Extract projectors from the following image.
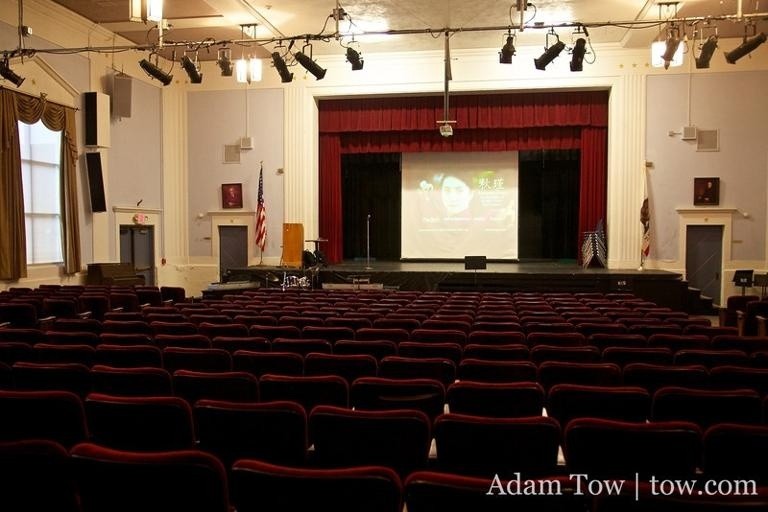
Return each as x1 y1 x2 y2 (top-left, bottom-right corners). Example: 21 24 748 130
440 125 454 136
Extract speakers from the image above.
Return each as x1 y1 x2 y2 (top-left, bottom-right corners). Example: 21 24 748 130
113 76 133 118
464 255 487 269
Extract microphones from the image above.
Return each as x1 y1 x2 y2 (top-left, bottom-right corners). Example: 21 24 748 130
366 214 370 223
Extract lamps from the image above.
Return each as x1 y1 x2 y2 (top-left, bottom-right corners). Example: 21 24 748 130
125 0 166 24
653 2 689 70
232 20 267 83
0 15 768 89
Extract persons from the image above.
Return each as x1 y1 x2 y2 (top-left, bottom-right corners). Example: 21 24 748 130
697 181 713 203
224 187 240 207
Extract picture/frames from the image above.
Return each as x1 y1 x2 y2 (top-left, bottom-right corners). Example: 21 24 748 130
692 175 721 207
221 182 244 211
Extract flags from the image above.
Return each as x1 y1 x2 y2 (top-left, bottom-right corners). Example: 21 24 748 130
638 164 651 264
253 164 266 252
